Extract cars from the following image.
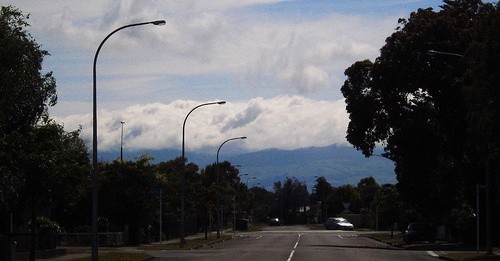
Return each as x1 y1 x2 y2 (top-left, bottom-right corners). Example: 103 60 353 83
325 217 354 231
269 218 281 227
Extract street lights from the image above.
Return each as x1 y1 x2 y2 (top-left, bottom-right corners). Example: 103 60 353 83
180 101 226 245
233 165 260 236
216 136 248 240
91 19 167 261
